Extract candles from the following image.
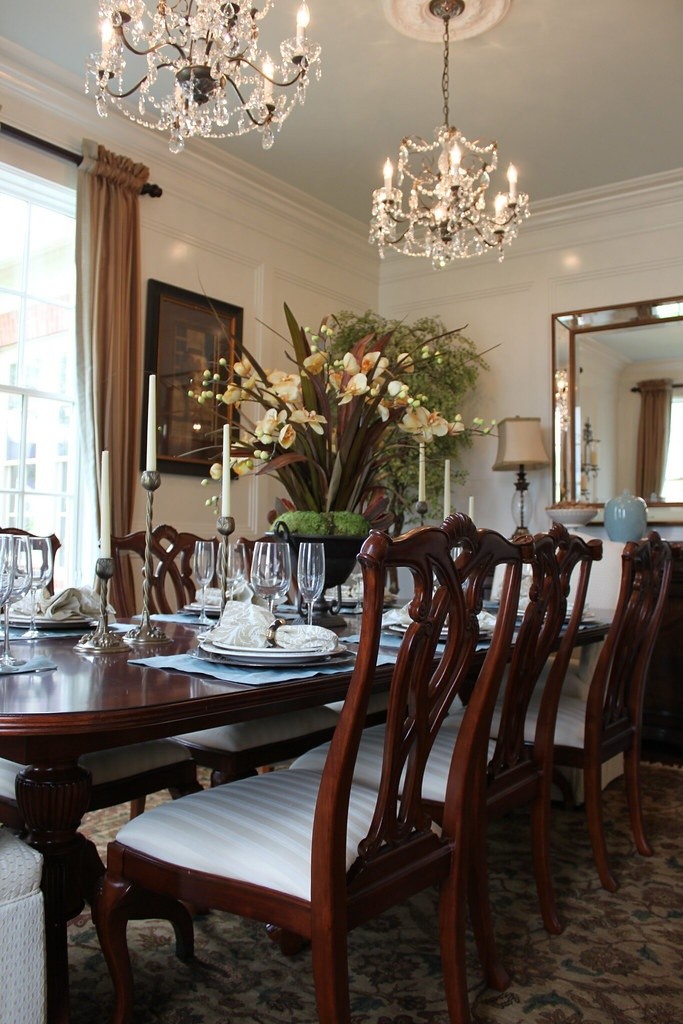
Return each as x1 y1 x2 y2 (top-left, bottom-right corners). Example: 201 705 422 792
220 423 233 519
101 450 113 560
443 459 453 521
468 496 474 523
145 375 157 475
418 440 428 506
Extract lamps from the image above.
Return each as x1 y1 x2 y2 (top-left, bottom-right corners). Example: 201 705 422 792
82 0 322 155
370 0 531 266
492 415 549 548
579 418 601 502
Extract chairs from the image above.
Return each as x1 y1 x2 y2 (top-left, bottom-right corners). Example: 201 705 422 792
293 524 601 996
236 532 390 728
101 515 515 1024
459 531 672 895
107 525 342 821
2 528 200 828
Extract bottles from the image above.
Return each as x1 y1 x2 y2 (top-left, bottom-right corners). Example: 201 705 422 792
603 489 649 543
250 541 291 613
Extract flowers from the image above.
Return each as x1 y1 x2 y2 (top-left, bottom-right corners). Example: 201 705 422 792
198 301 500 513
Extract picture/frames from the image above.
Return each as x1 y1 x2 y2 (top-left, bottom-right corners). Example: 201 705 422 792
142 278 243 482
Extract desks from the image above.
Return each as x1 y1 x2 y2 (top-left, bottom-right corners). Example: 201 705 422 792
0 598 615 1024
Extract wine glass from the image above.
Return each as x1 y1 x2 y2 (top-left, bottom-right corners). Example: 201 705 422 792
215 542 247 628
296 542 325 626
350 560 364 613
21 537 54 639
0 533 19 673
0 535 34 666
193 541 215 623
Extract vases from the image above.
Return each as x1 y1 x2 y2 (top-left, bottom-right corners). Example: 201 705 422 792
265 511 369 629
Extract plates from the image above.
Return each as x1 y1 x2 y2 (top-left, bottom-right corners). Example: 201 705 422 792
388 623 492 641
517 608 585 619
188 641 356 667
184 602 279 616
8 613 99 629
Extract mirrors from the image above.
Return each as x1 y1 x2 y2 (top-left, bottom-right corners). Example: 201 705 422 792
547 295 683 526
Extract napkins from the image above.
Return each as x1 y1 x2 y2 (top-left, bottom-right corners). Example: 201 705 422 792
209 599 336 652
13 588 115 623
196 582 255 614
500 572 591 621
381 595 496 632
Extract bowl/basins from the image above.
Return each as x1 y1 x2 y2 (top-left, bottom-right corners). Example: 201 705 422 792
545 509 598 528
